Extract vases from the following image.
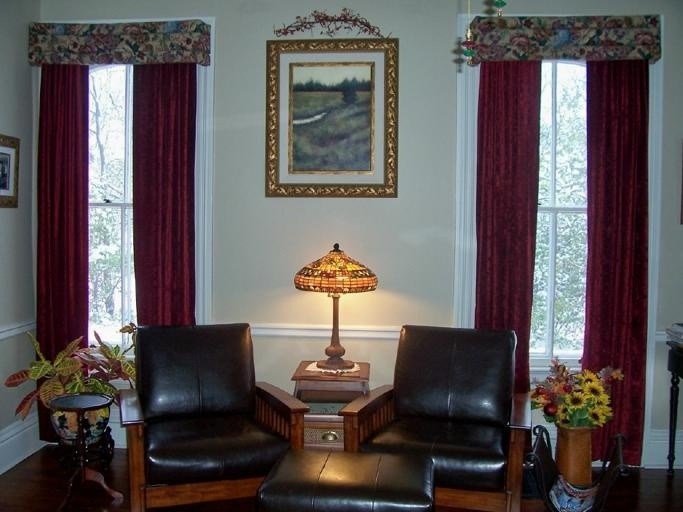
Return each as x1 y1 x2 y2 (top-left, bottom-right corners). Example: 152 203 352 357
555 425 593 486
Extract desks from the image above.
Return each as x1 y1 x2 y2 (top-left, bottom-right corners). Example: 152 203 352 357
665 342 683 474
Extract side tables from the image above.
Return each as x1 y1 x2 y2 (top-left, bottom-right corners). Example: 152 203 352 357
49 392 124 512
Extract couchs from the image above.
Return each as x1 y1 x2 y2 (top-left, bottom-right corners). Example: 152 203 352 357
337 325 532 512
257 448 434 512
118 323 310 512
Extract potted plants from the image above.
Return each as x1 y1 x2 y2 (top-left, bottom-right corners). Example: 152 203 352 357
3 321 136 450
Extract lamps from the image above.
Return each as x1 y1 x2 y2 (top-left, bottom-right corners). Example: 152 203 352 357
294 242 378 370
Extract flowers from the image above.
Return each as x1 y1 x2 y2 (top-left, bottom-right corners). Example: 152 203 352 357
527 356 625 427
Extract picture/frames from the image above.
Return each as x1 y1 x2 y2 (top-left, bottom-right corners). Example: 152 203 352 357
0 134 21 208
264 38 397 198
